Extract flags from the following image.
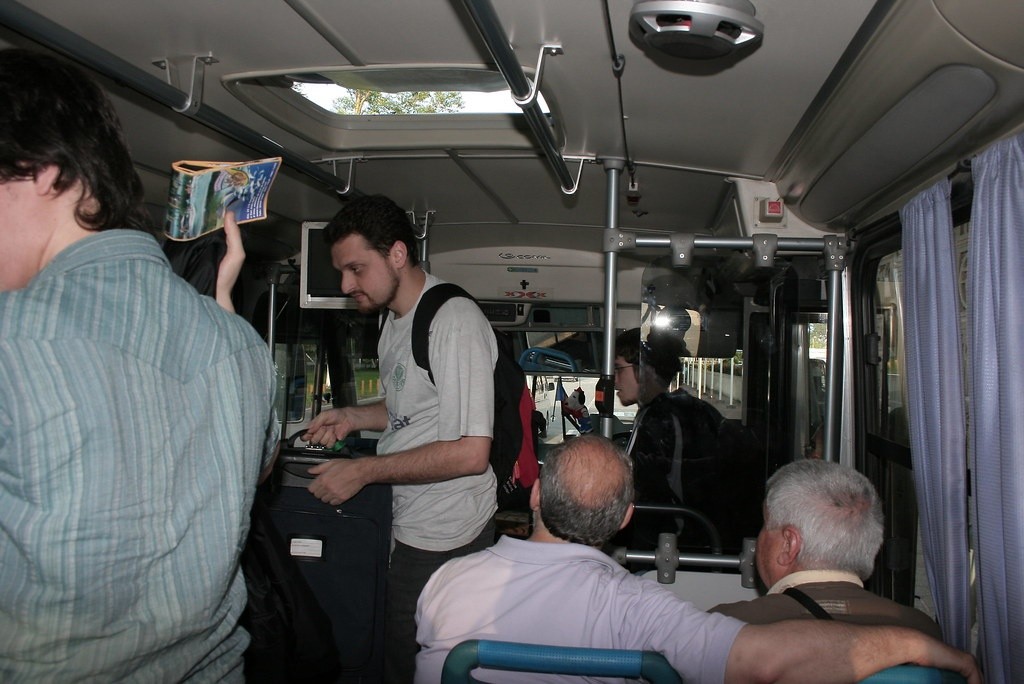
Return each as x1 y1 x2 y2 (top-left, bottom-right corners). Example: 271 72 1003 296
556 378 569 401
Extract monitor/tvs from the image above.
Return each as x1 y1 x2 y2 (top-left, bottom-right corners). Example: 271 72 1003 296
300 221 361 309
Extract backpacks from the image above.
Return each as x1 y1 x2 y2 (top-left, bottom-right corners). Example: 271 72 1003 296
374 283 547 487
636 390 765 555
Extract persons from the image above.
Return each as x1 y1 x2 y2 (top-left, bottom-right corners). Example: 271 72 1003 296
705 460 944 645
808 419 830 459
414 434 982 683
0 48 278 683
613 328 722 533
302 194 500 600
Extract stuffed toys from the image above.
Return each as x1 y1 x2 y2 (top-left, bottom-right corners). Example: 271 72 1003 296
562 386 593 432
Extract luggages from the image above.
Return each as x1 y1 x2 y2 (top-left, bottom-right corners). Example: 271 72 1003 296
242 429 391 684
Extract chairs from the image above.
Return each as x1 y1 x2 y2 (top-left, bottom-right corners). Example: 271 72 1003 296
519 346 576 372
441 640 971 683
